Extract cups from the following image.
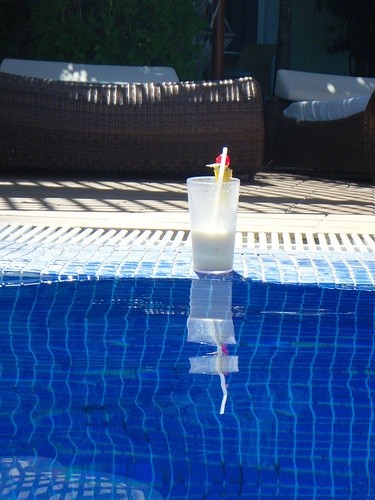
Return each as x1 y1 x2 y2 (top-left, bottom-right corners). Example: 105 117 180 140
187 175 241 275
185 280 242 379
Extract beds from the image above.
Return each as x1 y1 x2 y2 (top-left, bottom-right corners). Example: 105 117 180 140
267 70 373 181
1 57 262 183
